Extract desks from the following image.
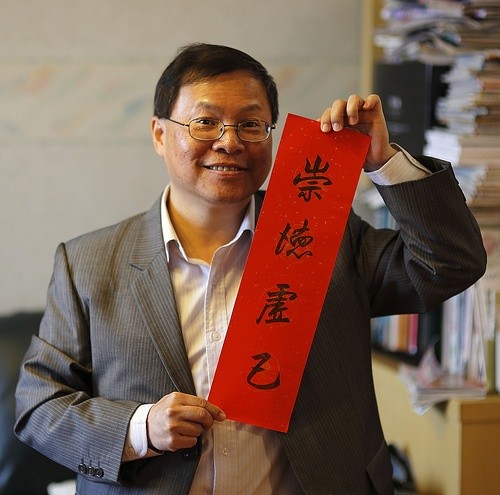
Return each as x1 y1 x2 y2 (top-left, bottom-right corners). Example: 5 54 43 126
363 344 500 495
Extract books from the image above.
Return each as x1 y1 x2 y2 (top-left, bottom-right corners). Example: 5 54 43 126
361 1 499 415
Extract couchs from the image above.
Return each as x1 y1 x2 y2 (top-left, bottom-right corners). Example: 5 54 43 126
1 310 79 495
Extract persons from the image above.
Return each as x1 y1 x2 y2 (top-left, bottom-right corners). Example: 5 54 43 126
13 44 487 495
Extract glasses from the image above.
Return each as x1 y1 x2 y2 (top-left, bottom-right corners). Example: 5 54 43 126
159 113 276 144
